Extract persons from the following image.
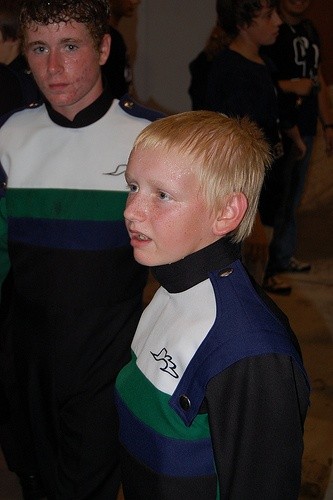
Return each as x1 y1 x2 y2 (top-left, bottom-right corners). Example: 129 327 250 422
0 0 169 500
189 0 327 294
112 109 311 500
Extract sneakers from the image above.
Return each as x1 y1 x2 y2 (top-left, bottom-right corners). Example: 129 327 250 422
267 256 311 272
262 270 292 295
